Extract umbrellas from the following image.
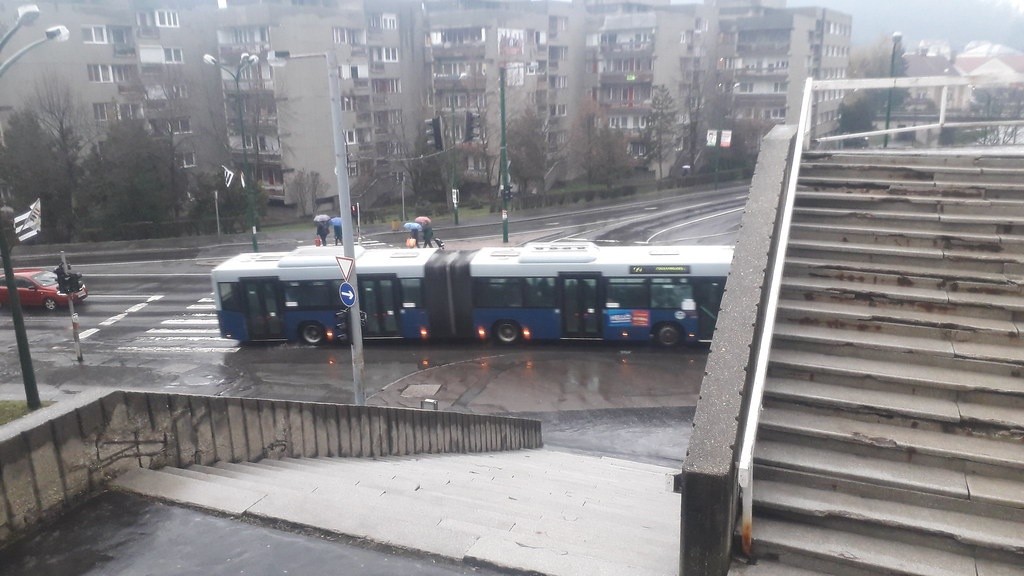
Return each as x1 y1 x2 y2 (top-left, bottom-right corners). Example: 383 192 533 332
415 216 431 226
329 218 342 226
314 214 330 222
403 223 422 231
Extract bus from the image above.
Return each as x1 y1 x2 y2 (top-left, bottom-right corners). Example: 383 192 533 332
211 241 736 347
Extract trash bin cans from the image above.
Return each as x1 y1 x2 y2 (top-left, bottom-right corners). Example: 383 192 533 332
392 220 400 231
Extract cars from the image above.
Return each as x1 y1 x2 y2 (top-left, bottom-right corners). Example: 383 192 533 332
0 270 88 312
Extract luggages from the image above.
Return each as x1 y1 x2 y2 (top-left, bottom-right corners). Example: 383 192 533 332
432 237 445 249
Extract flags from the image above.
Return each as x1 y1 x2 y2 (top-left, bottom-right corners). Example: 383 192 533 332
13 200 41 242
223 169 233 187
240 173 246 187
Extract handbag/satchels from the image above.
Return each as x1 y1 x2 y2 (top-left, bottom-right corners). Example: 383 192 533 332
406 237 417 247
316 236 320 246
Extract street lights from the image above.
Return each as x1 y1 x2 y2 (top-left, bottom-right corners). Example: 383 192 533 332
204 53 261 253
499 62 539 243
883 32 903 147
0 4 71 409
267 50 367 406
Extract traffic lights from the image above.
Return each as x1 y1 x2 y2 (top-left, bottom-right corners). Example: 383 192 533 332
53 268 72 294
70 273 83 292
466 111 480 141
424 117 442 150
508 185 513 201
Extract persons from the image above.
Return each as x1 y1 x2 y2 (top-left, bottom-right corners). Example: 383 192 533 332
707 133 715 146
317 221 330 246
422 223 433 248
410 229 420 248
334 225 343 246
